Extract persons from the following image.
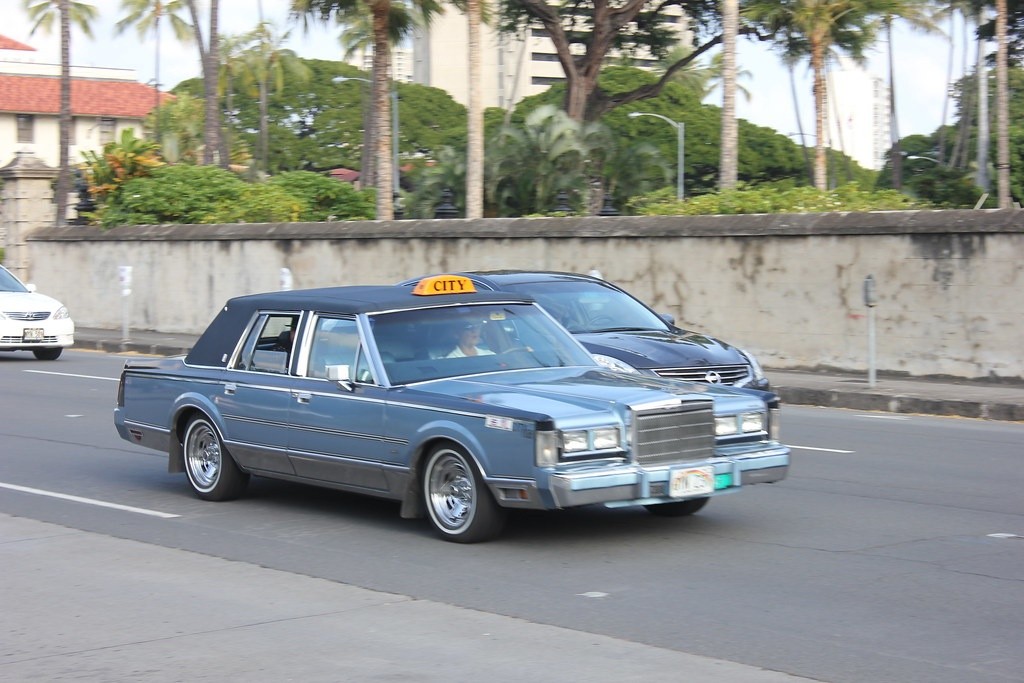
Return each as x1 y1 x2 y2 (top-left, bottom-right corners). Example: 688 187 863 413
445 316 495 358
273 314 323 373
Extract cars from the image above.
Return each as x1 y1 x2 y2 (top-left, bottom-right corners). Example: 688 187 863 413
0 263 76 359
327 266 771 394
114 271 790 544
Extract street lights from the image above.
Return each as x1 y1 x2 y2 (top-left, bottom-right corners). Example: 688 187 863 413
332 75 402 221
626 112 685 207
905 154 944 204
787 131 837 192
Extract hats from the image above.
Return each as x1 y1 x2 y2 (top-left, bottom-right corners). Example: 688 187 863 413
284 315 327 332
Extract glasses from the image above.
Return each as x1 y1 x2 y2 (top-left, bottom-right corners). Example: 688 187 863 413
462 320 487 331
